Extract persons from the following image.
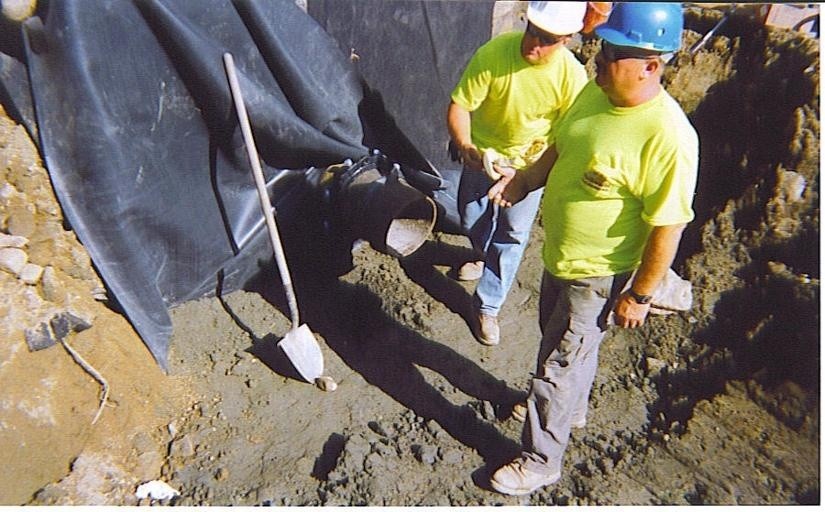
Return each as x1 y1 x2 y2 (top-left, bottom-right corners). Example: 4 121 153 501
443 1 589 347
489 0 698 495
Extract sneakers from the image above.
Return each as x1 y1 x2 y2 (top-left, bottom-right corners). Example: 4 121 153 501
487 457 561 495
511 401 586 428
464 313 499 345
458 260 485 281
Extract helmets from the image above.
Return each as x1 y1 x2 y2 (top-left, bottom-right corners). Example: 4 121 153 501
593 2 684 52
527 1 587 35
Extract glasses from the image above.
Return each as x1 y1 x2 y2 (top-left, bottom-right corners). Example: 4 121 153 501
528 23 561 45
602 39 651 61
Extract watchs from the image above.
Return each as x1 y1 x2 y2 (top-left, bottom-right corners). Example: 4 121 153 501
630 288 654 308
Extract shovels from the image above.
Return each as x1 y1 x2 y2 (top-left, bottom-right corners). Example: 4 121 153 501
223 52 324 386
